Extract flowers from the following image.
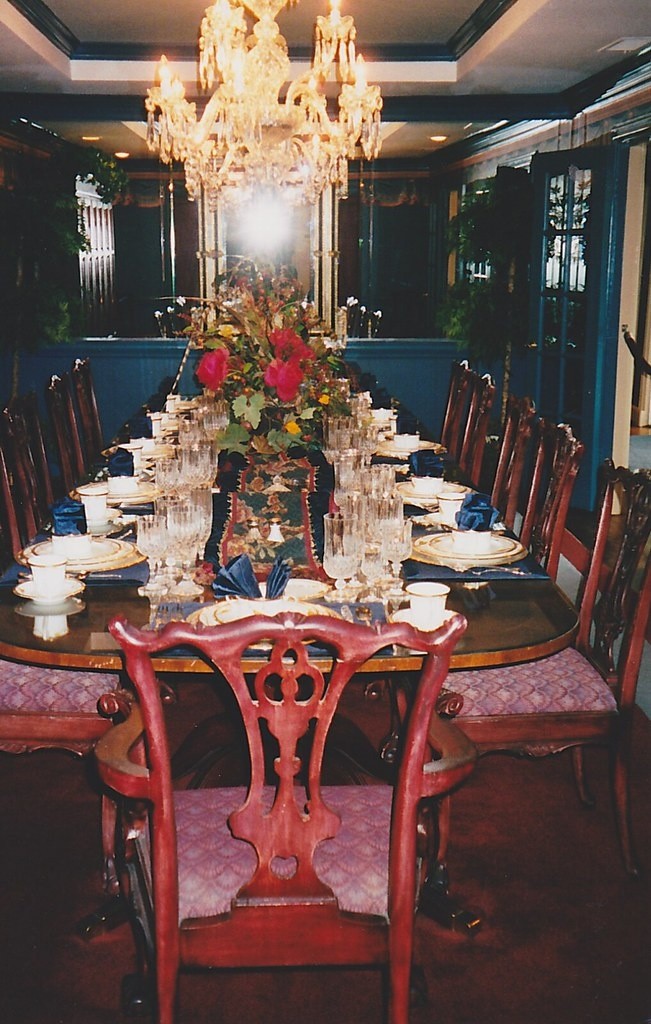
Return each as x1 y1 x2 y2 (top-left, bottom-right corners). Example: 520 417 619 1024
148 249 383 454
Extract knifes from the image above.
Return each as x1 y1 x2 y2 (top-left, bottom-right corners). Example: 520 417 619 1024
342 605 354 624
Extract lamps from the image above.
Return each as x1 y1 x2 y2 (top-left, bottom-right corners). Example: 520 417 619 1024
146 0 383 213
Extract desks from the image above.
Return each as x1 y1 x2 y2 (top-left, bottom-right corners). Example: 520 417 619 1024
0 393 579 672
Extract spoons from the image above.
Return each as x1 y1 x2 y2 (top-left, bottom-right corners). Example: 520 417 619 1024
356 606 373 627
18 570 90 580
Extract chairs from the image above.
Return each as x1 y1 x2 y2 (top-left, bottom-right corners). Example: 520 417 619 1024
0 356 651 1024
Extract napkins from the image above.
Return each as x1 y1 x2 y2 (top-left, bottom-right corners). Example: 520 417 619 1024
212 553 292 602
107 448 134 475
149 602 394 658
457 491 498 530
402 527 551 579
49 493 87 536
0 534 149 587
409 449 454 476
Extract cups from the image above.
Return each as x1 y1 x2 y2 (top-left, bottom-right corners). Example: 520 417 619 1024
130 439 156 454
108 476 139 493
27 555 67 595
51 533 92 560
452 530 491 553
166 394 176 412
436 493 466 521
412 478 445 494
79 488 109 519
151 414 162 437
406 582 450 623
370 410 393 419
394 435 420 449
118 444 143 466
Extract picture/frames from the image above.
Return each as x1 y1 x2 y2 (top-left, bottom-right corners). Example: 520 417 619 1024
197 179 338 335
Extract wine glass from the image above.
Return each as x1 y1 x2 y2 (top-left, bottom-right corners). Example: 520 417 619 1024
333 427 395 507
153 495 182 577
136 516 167 596
166 504 205 595
367 491 404 579
344 494 362 586
155 409 230 493
191 488 212 566
382 519 413 600
323 513 358 603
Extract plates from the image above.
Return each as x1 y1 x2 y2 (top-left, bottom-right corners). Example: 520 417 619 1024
171 401 196 410
76 510 123 525
188 599 341 649
252 579 328 601
378 440 443 457
389 608 468 632
409 533 526 567
100 443 176 458
70 479 157 506
15 537 147 570
397 481 471 505
13 580 86 603
